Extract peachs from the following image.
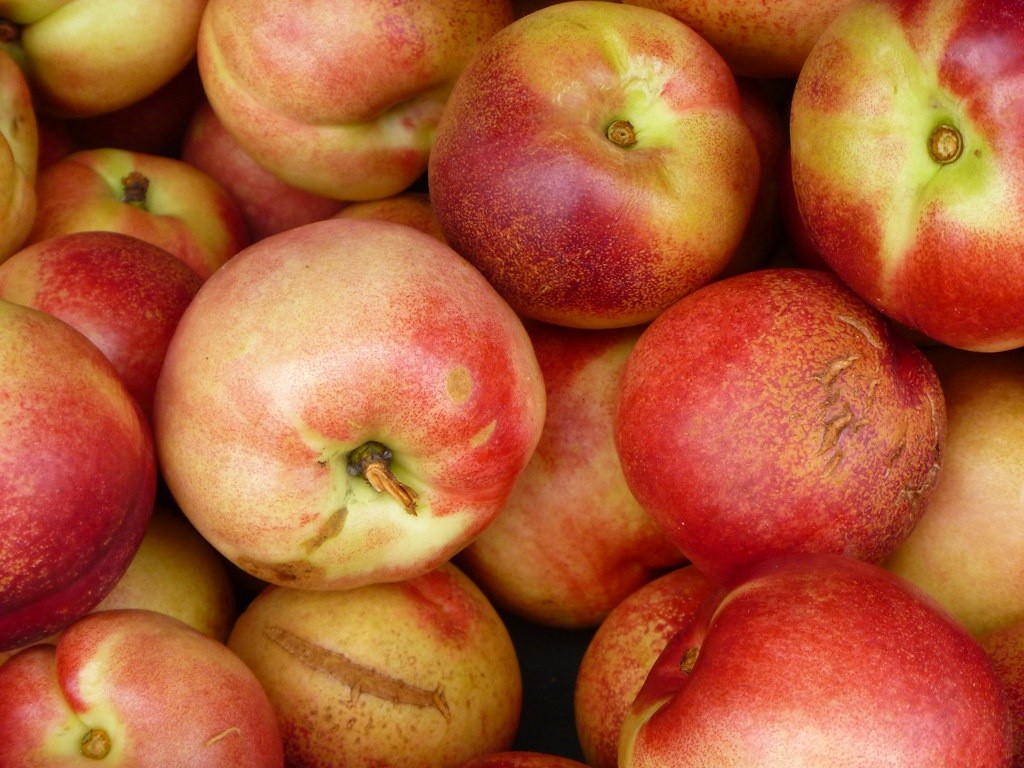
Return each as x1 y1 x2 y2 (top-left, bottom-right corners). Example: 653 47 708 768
0 0 1024 768
